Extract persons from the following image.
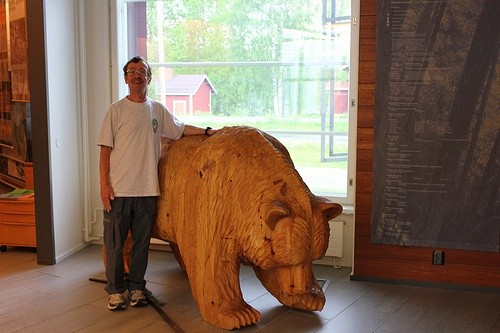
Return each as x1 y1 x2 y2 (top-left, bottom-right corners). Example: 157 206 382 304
96 55 222 311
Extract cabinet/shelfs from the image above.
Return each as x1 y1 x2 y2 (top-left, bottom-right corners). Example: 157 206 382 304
0 189 37 252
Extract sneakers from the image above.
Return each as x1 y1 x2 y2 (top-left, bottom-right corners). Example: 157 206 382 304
108 293 128 311
128 290 148 307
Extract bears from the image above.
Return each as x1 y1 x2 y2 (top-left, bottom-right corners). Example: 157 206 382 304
102 125 343 330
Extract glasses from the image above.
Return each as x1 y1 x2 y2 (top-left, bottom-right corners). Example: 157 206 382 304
126 70 149 74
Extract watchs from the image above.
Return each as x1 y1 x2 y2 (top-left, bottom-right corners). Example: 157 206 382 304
205 126 212 136
